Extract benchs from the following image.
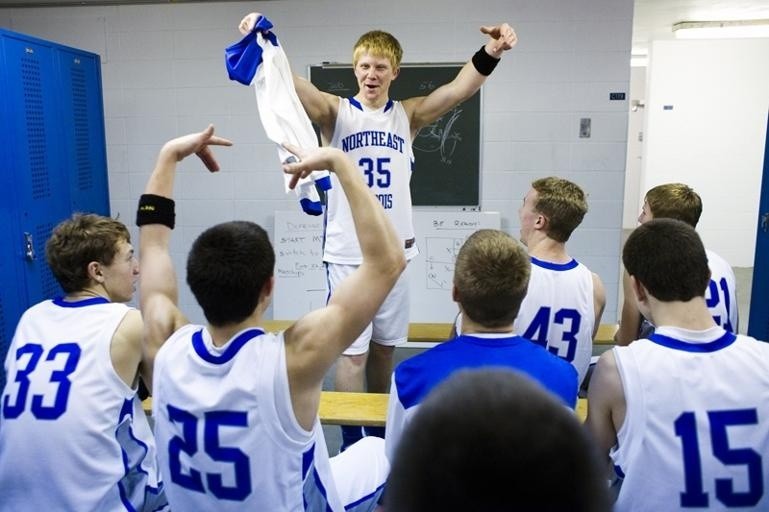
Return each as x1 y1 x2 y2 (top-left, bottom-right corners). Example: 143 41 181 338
141 391 590 428
257 316 621 345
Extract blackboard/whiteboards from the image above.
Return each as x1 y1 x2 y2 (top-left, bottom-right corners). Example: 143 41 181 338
273 210 501 323
307 62 483 211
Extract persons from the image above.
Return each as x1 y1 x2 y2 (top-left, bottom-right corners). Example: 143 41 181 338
237 11 518 451
385 228 579 466
578 182 739 398
0 208 172 512
449 177 606 402
382 365 615 512
584 217 769 512
134 124 408 512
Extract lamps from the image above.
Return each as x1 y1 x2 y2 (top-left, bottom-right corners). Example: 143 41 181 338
671 19 769 39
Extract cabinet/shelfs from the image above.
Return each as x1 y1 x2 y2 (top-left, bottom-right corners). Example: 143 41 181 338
0 30 112 396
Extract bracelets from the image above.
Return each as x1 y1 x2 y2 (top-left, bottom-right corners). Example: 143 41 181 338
471 44 502 76
136 193 176 229
